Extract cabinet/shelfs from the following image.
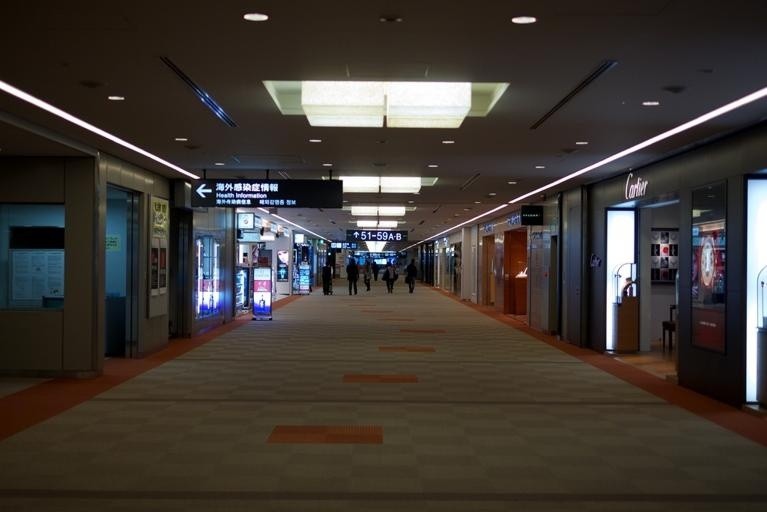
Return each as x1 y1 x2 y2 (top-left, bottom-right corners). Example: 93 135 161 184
610 260 642 353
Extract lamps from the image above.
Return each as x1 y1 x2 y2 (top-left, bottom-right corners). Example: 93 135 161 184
339 174 422 194
351 205 406 217
301 79 473 128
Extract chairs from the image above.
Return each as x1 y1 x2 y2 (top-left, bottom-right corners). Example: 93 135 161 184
661 304 677 353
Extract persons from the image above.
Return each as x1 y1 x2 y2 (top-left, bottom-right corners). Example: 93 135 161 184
381 262 396 294
363 262 370 291
322 261 333 295
403 258 417 293
356 261 402 277
372 262 378 281
346 259 359 295
277 251 287 265
